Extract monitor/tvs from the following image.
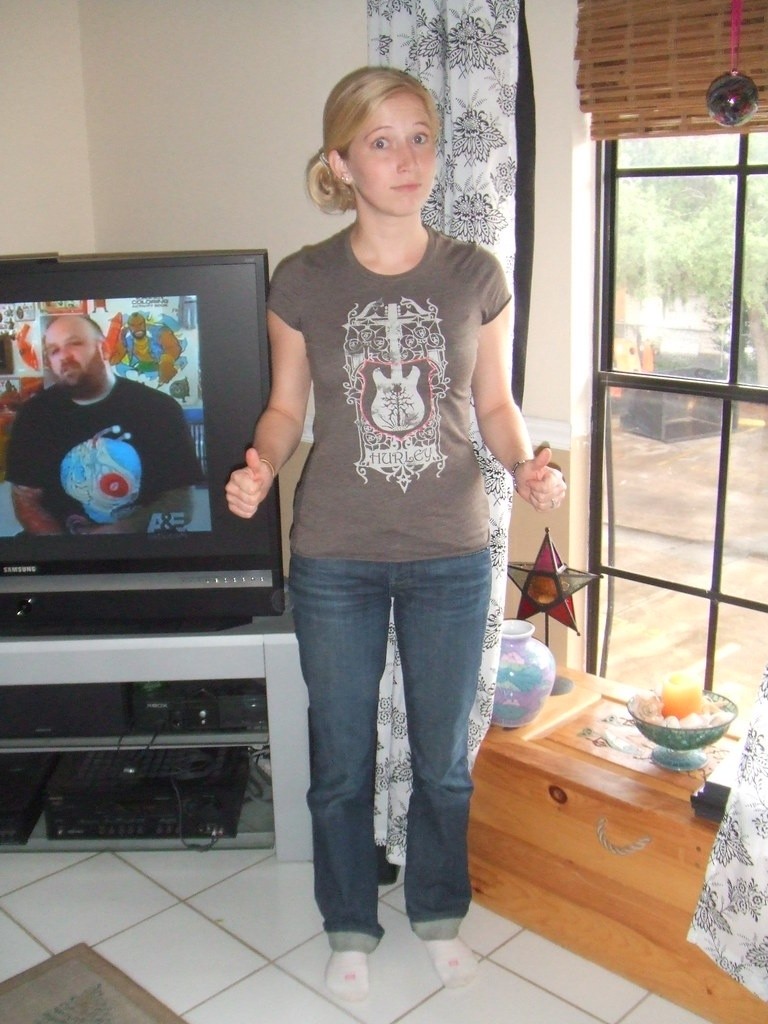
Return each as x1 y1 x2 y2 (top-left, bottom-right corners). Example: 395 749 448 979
0 249 287 637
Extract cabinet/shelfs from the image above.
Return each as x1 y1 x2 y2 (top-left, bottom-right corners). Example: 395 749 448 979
0 581 313 862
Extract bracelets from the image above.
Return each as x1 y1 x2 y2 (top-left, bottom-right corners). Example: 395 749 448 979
511 459 526 493
258 458 275 477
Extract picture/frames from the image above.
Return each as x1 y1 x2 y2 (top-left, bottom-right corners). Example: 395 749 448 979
43 300 86 313
0 333 14 375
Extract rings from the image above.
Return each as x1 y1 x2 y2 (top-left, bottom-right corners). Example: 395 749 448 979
551 498 559 508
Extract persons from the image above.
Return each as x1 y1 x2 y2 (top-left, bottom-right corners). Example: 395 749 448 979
224 66 572 996
4 314 201 539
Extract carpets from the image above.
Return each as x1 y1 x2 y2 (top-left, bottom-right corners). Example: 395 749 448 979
0 943 189 1024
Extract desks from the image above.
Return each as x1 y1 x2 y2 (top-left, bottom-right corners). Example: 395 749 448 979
468 665 768 1024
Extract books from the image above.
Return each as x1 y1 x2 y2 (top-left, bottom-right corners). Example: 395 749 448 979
689 734 747 824
